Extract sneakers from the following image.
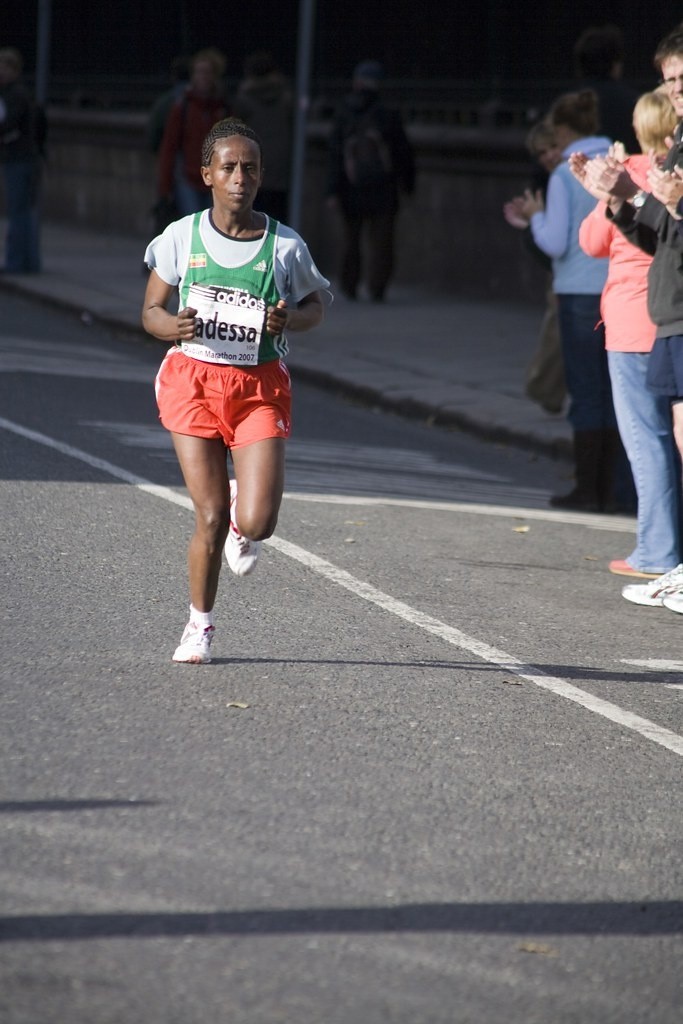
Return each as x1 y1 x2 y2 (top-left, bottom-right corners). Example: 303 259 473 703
171 620 216 666
663 589 683 613
225 478 260 576
621 564 683 606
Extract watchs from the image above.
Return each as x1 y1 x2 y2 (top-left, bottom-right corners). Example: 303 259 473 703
631 191 649 210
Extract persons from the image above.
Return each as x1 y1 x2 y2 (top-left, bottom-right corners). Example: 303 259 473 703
0 48 50 275
502 23 683 614
141 117 330 664
142 47 235 273
324 62 418 303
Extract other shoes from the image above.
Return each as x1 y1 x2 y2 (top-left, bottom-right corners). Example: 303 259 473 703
609 560 664 578
547 488 637 514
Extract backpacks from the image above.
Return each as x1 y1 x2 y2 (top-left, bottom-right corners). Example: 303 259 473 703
339 108 398 190
